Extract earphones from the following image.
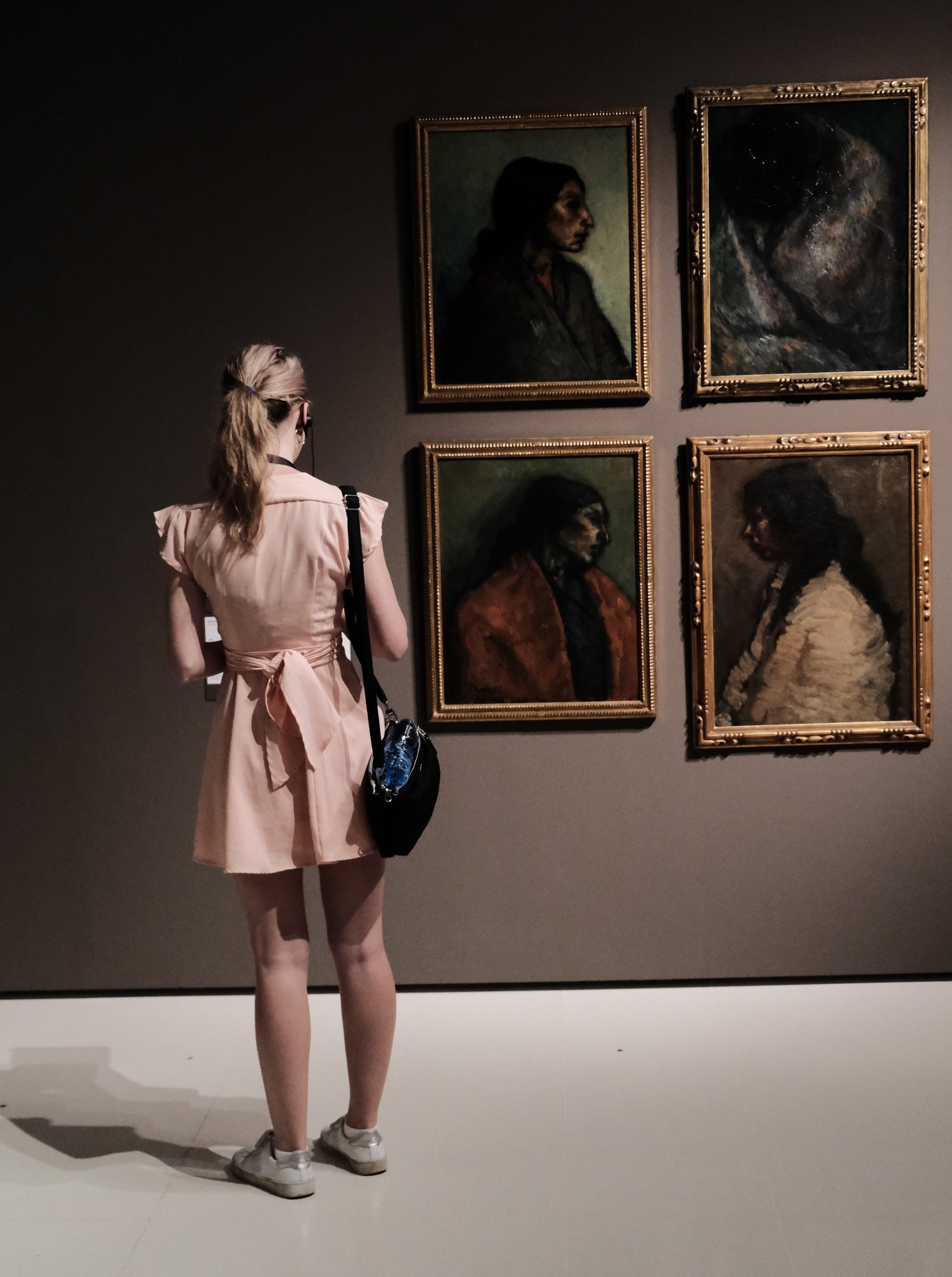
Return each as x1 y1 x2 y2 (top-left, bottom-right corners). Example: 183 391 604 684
304 414 313 430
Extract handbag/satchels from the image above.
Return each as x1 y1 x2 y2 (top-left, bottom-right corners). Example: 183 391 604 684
361 718 442 859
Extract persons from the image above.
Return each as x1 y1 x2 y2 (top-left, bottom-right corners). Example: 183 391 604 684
152 344 409 1201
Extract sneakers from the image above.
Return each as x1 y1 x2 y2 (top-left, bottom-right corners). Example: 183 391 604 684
321 1113 387 1176
232 1129 317 1198
